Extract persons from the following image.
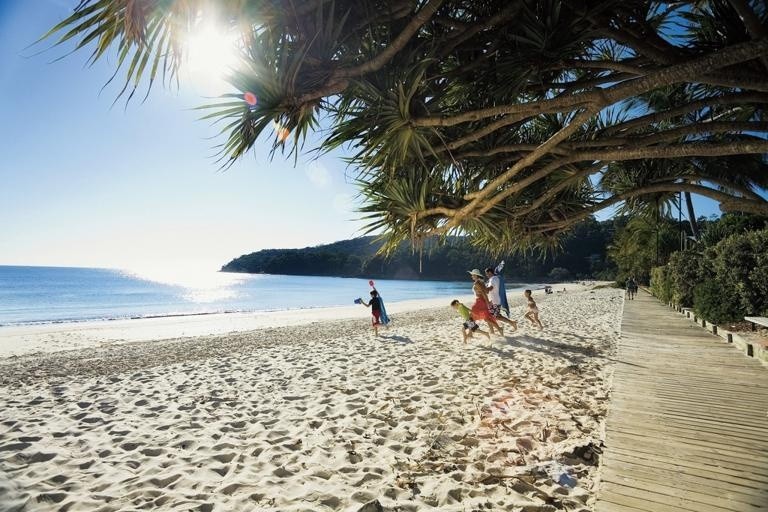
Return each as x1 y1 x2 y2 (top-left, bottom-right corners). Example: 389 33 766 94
467 269 504 337
359 290 388 337
451 300 490 345
484 268 518 334
524 290 543 331
634 279 638 293
632 276 635 284
626 277 635 300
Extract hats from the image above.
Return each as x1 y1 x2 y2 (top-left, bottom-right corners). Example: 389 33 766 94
466 268 485 279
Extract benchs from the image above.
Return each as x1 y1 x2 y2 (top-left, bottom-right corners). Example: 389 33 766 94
743 316 768 332
